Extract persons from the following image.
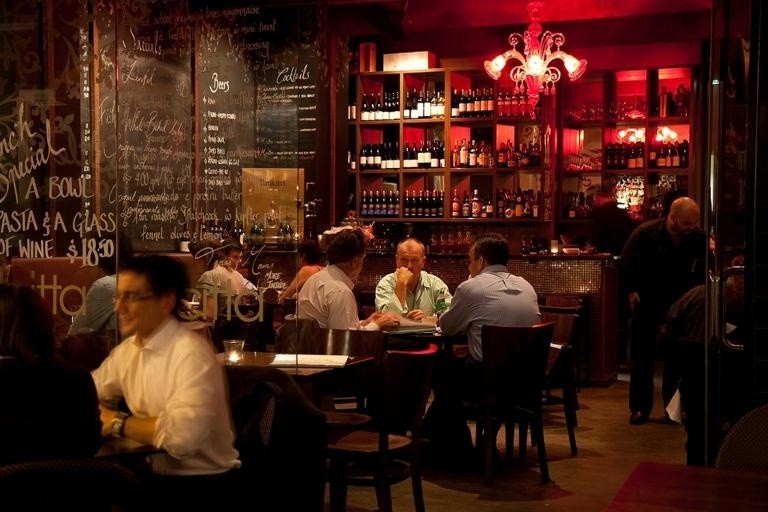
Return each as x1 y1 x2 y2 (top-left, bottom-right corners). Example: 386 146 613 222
195 240 267 352
665 247 744 466
582 185 642 372
618 198 715 426
0 283 105 467
437 234 541 476
280 240 326 299
297 228 400 395
375 239 453 436
90 256 253 512
0 252 11 285
67 230 135 370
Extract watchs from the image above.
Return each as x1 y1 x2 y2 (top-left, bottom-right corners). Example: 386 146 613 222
111 412 129 437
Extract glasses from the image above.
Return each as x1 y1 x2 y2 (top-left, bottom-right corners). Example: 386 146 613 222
111 289 151 304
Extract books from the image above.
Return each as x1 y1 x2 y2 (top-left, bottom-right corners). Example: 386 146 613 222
270 354 356 368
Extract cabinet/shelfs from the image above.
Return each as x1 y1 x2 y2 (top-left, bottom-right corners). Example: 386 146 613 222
355 72 550 253
552 67 701 253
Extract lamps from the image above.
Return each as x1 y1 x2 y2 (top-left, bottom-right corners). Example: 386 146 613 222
618 93 678 145
484 17 588 121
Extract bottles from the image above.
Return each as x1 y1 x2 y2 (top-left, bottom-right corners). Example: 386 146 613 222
451 88 459 117
368 93 375 121
438 189 444 218
387 190 394 218
435 288 447 319
430 89 438 120
394 91 400 120
473 88 480 119
656 140 665 167
439 140 446 169
628 142 637 168
374 190 380 218
512 91 518 117
424 90 431 120
496 139 542 169
480 88 488 117
437 91 444 119
679 140 688 168
404 90 410 119
613 142 620 170
387 142 394 170
672 141 680 168
519 91 526 117
636 141 644 168
380 190 387 219
403 141 410 169
466 89 474 118
620 142 627 169
410 189 417 218
665 141 672 167
417 90 424 119
459 138 467 168
424 140 431 169
394 189 400 218
417 140 424 168
471 188 480 219
380 143 386 170
417 189 424 218
423 189 430 218
351 105 356 120
504 90 512 117
411 142 418 169
459 89 467 119
478 141 488 168
375 93 382 120
451 139 459 169
360 94 368 120
389 93 395 120
367 190 374 219
487 87 494 118
497 91 503 117
361 190 367 218
366 143 374 170
394 140 400 170
461 190 471 219
451 188 460 218
346 105 351 119
496 186 542 219
429 189 437 218
486 190 494 219
410 88 419 120
382 92 389 120
374 143 381 169
649 141 656 167
403 189 410 218
468 140 477 168
431 142 438 168
359 142 366 170
605 142 613 170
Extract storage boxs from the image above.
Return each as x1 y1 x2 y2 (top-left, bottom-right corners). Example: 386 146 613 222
383 50 436 73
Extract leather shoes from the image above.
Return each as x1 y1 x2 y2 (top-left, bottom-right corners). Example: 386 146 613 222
629 410 648 424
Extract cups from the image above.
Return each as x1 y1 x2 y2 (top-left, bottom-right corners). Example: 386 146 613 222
222 340 245 368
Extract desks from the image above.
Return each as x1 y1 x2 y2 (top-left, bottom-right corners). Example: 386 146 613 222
215 345 378 410
380 324 467 358
603 460 768 512
91 404 168 512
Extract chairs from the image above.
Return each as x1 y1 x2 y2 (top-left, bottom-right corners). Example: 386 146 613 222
0 461 149 511
325 343 440 512
312 327 383 429
502 333 593 471
441 321 555 484
